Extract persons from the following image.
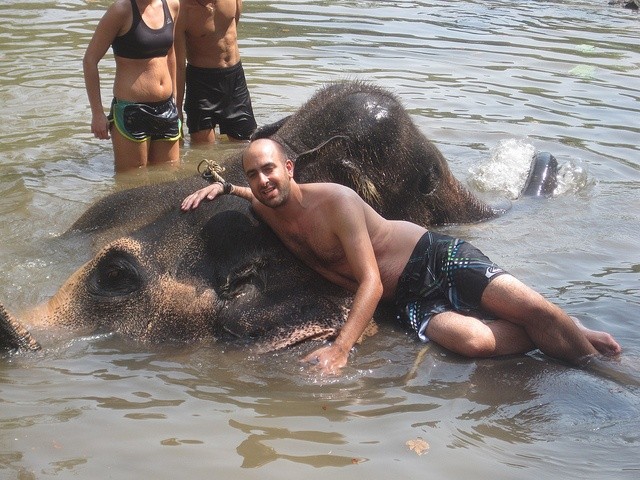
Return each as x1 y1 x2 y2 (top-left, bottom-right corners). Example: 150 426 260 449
82 1 181 169
175 0 257 143
181 139 622 379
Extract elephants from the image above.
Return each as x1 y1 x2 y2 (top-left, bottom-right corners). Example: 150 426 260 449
3 80 559 372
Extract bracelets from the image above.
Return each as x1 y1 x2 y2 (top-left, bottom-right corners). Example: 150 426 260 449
223 181 231 195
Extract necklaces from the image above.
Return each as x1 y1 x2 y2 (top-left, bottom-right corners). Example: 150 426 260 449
197 0 215 14
